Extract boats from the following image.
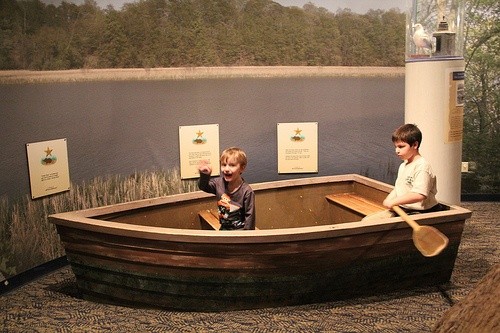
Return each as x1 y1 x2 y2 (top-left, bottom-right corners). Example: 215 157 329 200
46 172 473 311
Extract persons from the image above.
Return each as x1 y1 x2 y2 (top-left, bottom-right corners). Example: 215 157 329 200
359 123 440 222
198 147 256 230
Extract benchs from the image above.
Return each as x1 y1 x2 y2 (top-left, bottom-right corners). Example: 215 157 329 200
199 209 260 231
325 194 390 216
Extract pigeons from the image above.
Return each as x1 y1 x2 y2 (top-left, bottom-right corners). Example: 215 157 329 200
412 24 433 55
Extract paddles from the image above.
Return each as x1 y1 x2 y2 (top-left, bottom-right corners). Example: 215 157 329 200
391 204 449 258
